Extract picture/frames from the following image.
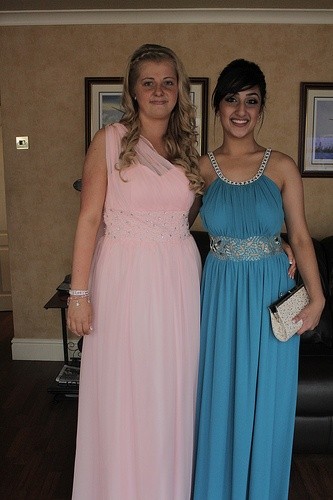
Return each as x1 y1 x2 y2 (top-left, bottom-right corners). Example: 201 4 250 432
298 81 333 178
85 76 209 159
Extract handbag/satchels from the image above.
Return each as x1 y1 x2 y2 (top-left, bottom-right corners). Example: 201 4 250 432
267 282 310 343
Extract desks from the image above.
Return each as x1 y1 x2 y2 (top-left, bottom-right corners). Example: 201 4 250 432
44 273 83 400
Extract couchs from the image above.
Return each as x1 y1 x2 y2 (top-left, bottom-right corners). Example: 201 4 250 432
191 231 333 452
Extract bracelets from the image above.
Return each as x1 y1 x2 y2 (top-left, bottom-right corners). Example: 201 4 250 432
67 289 91 307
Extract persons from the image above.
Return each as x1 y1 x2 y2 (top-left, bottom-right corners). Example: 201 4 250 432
187 58 326 500
66 44 298 500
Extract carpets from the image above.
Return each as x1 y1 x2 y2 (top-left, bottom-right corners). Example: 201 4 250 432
296 455 333 500
